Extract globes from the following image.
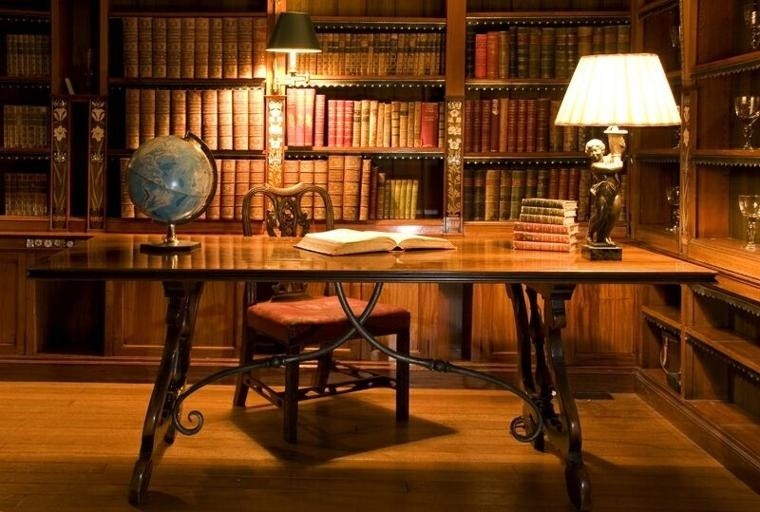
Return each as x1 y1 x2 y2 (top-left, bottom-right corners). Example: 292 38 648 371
125 130 218 252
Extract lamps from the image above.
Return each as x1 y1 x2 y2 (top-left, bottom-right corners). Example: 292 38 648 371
265 13 322 80
554 52 681 260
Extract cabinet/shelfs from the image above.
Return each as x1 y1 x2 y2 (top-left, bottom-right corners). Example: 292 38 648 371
636 0 760 497
1 0 638 394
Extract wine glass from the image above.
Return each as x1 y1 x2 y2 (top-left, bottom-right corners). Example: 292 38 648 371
734 95 760 150
669 25 679 69
672 125 680 150
742 3 759 50
737 195 759 251
666 185 680 233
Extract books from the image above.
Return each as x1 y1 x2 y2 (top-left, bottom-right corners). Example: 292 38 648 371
122 17 267 223
512 196 579 253
294 226 457 256
284 2 445 222
3 106 50 152
464 1 633 220
5 35 51 78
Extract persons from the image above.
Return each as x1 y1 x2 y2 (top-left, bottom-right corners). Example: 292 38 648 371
4 173 48 215
580 138 626 248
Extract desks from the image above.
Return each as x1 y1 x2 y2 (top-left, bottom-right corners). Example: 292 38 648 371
27 233 718 512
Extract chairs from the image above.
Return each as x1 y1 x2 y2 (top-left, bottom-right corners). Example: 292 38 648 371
234 183 410 443
